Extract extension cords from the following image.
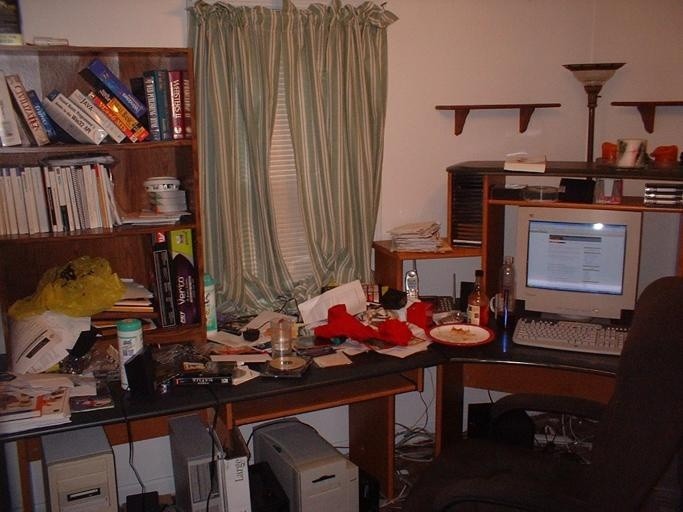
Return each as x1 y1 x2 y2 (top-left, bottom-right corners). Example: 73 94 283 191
534 434 575 447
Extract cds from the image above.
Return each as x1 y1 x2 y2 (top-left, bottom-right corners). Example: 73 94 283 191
526 186 558 201
270 356 307 370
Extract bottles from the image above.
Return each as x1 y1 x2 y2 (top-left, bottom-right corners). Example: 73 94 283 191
269 317 293 359
498 256 516 327
202 274 218 338
116 318 143 392
466 270 491 327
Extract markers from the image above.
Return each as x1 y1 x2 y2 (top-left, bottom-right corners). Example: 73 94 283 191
175 376 232 385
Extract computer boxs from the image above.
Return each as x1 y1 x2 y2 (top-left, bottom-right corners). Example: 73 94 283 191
39 426 119 511
253 417 360 512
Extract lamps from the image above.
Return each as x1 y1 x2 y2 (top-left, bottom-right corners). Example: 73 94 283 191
563 63 627 180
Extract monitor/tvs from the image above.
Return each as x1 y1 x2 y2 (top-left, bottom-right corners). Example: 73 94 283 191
514 204 643 321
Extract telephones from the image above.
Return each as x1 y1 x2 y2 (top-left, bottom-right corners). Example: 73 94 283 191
405 259 456 312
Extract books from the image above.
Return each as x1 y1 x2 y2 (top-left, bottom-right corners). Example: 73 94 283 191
91 229 195 329
1 153 192 236
1 58 191 146
502 152 548 174
0 371 114 435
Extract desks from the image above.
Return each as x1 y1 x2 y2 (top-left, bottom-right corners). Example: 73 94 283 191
427 318 621 458
0 345 450 512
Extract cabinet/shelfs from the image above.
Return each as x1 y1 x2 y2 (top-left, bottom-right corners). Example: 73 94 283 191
371 161 683 314
0 45 207 370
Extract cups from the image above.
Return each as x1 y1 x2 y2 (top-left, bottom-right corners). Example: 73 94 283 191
489 294 513 320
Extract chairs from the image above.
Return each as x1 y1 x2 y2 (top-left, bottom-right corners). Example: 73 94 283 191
421 275 683 512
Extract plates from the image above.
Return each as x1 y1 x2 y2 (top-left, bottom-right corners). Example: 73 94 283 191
425 323 496 346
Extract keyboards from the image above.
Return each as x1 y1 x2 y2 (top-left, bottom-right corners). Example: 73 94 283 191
512 317 631 357
